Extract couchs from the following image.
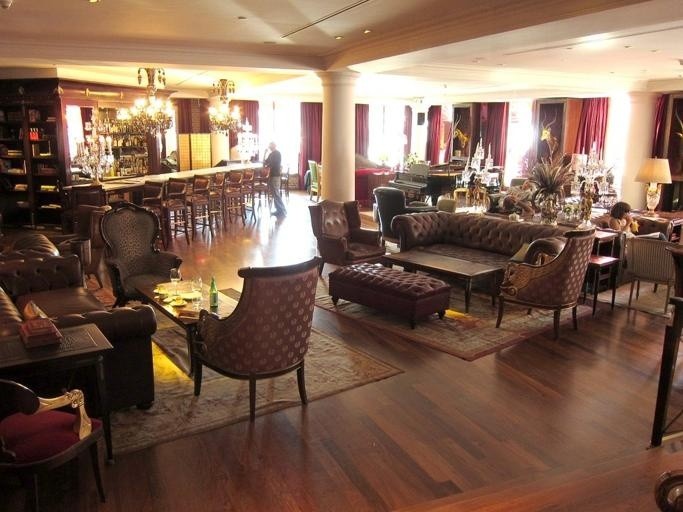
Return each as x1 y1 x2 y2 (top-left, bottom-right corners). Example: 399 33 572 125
390 209 565 269
1 232 158 411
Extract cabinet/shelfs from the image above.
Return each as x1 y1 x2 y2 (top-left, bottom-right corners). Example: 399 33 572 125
95 107 149 176
0 77 64 226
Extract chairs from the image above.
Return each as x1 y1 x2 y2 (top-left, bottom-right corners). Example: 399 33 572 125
0 378 106 512
624 238 676 316
495 228 595 335
308 158 321 203
308 201 386 265
62 168 290 251
372 187 438 246
193 255 323 423
99 202 184 308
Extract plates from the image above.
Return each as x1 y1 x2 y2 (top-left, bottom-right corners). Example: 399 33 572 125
152 289 196 306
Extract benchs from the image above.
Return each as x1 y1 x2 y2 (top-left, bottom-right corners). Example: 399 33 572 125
329 262 451 330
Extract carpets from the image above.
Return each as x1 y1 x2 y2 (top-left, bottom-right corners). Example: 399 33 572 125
88 270 404 454
314 290 599 358
587 272 679 325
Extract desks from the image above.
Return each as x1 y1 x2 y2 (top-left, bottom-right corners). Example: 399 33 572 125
380 250 502 313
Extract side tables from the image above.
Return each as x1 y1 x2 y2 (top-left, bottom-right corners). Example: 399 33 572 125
1 323 115 461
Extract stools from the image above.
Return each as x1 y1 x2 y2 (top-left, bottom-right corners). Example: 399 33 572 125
589 251 621 317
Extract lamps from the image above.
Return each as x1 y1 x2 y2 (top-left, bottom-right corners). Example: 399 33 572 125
208 78 241 130
634 156 673 215
578 139 607 190
236 118 259 162
73 106 114 183
115 67 178 134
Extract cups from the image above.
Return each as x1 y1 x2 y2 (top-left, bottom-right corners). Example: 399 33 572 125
508 214 519 221
532 214 541 223
455 150 462 158
631 222 638 231
116 171 121 177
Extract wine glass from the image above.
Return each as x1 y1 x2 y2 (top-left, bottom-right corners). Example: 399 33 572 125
189 276 203 311
169 268 181 292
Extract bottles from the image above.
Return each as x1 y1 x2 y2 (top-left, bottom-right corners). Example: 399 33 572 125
110 167 114 177
209 276 218 308
108 119 144 148
28 127 44 140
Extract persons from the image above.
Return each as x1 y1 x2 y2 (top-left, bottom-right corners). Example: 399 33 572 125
607 202 668 247
264 142 287 216
499 179 532 201
498 196 535 218
